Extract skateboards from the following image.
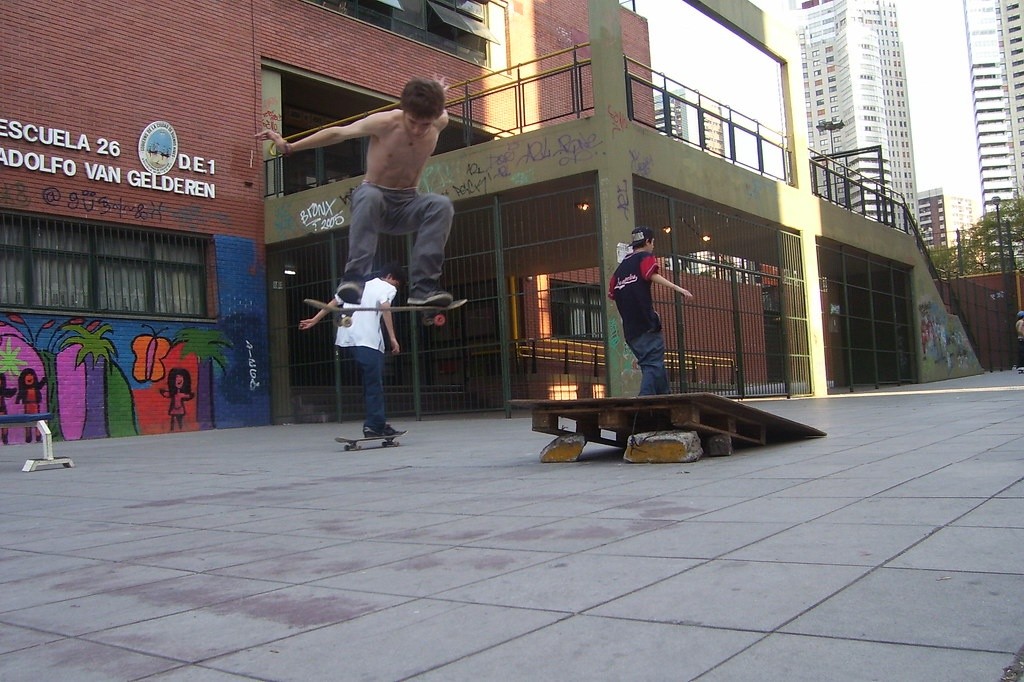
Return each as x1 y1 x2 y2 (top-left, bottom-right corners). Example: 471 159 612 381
334 430 409 451
1017 369 1024 374
304 298 468 328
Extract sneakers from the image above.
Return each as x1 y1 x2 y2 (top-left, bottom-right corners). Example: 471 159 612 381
363 424 385 438
337 274 365 305
407 278 453 307
384 424 399 435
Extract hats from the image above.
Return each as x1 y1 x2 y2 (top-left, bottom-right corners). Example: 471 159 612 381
627 225 651 247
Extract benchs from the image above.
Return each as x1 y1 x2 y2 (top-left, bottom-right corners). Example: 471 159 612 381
0 413 73 473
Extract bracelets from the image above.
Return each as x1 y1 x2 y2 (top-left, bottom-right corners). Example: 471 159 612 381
284 142 292 156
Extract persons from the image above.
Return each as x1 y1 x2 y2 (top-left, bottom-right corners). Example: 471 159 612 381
607 227 692 396
299 262 404 437
1015 311 1024 371
254 72 455 305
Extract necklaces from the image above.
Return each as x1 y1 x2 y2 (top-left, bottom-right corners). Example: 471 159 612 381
406 126 431 145
